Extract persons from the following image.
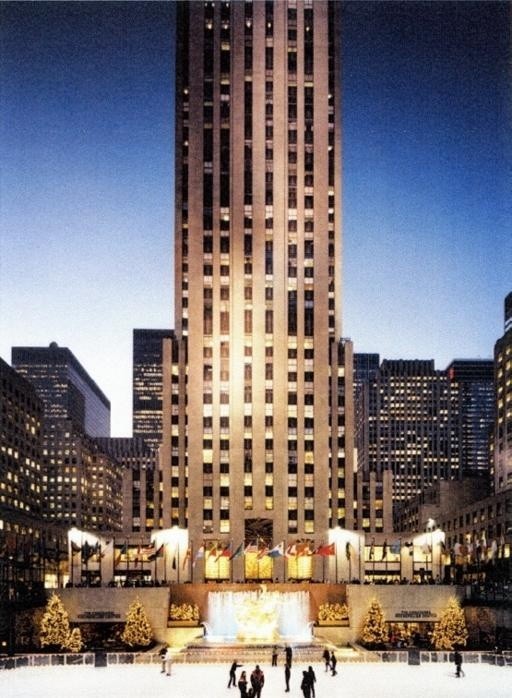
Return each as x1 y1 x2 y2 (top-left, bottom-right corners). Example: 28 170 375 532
64 573 457 588
272 645 278 667
228 658 243 688
387 630 433 650
330 651 337 676
307 666 317 698
284 646 292 669
322 649 332 673
454 652 465 677
251 665 264 698
238 671 247 698
245 688 256 698
160 652 171 676
301 671 312 698
284 664 290 692
158 648 168 673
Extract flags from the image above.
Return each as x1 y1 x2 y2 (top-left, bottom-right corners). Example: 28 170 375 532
13 535 504 566
